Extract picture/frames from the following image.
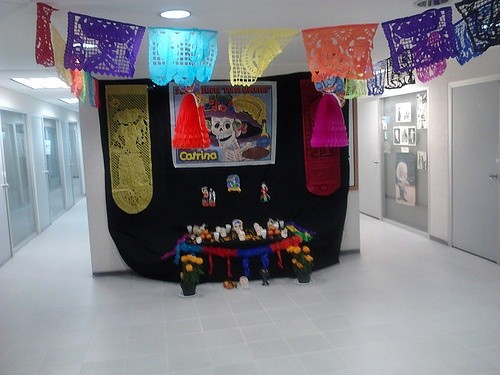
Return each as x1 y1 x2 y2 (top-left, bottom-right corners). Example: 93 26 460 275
382 92 427 207
